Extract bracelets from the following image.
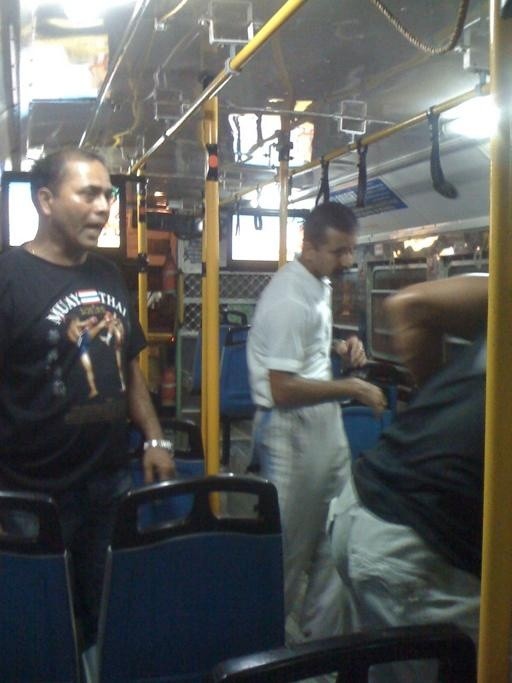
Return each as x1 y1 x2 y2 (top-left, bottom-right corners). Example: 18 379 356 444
143 437 175 454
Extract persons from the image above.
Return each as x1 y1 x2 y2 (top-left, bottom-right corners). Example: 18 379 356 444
101 310 128 395
242 199 392 641
1 145 179 655
66 309 110 400
324 268 488 681
